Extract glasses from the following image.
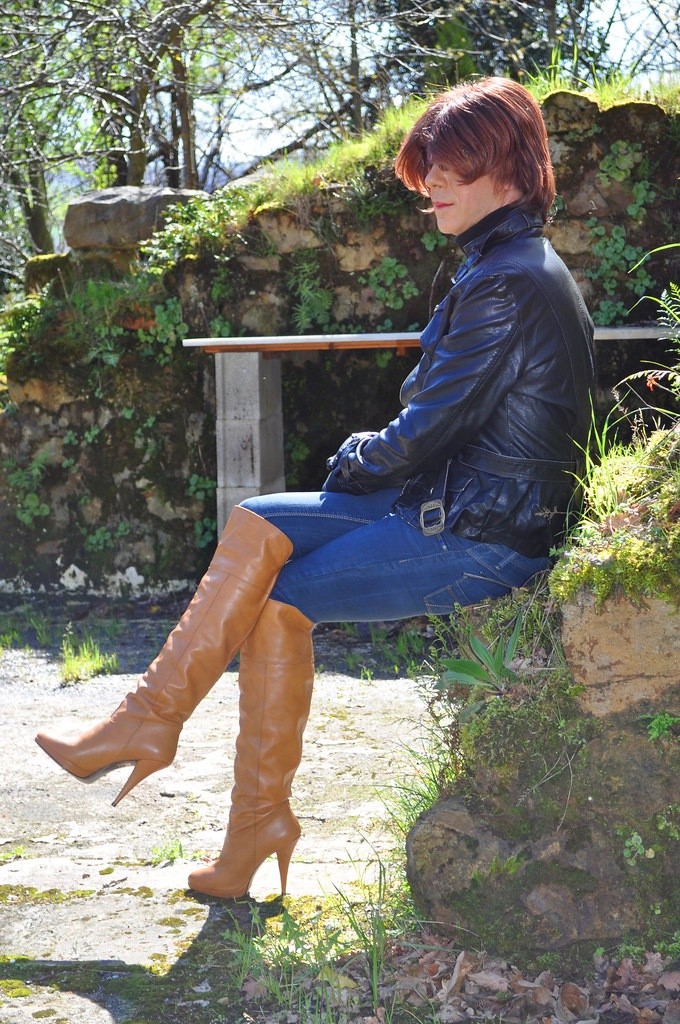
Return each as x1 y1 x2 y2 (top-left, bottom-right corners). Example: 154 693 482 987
427 149 471 171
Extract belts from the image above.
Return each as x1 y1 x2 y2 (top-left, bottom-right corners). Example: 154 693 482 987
420 445 588 537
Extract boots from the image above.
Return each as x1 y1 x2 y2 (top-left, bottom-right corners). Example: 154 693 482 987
188 599 316 898
34 505 295 807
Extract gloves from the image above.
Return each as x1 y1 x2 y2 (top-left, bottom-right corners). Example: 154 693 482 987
321 469 350 493
326 430 378 470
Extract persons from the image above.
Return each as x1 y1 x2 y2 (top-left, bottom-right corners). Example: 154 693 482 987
34 76 597 900
